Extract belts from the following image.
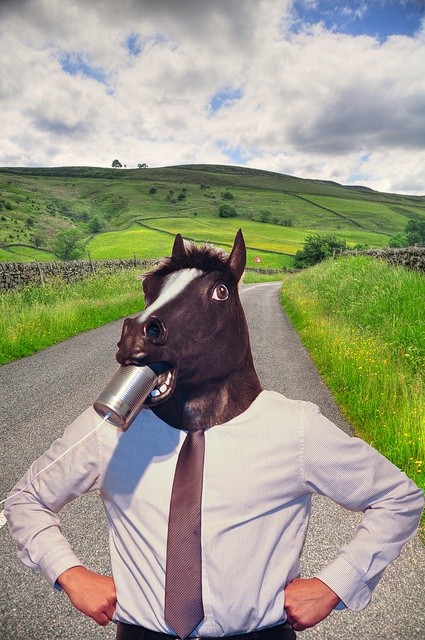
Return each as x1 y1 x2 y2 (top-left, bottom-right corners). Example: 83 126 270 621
114 622 295 640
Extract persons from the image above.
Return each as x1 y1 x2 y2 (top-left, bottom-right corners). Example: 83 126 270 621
4 402 425 640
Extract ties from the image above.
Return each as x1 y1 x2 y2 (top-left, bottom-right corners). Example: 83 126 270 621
164 430 205 639
